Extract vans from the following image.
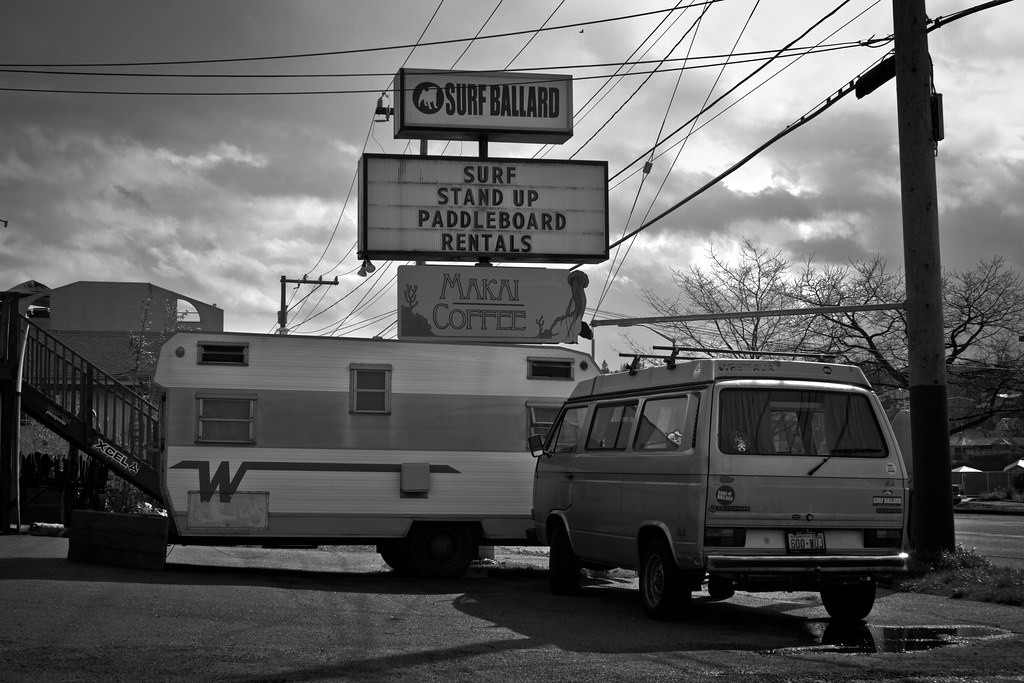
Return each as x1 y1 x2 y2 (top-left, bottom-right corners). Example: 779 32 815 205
527 346 910 623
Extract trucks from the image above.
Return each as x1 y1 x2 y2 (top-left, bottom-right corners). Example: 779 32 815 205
150 330 603 591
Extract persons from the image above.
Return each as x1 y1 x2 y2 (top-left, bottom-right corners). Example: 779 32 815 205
1016 478 1024 495
